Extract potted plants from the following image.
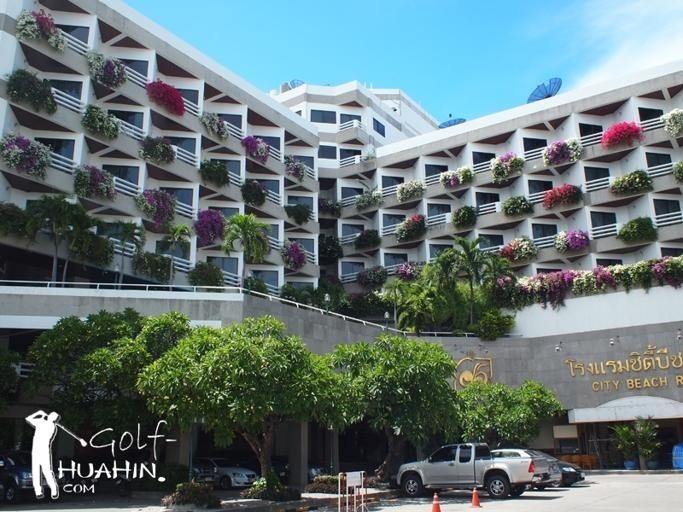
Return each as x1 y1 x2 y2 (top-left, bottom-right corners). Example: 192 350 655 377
633 414 663 470
606 423 640 470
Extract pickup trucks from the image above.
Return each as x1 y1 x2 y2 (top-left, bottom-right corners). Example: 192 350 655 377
396 442 550 499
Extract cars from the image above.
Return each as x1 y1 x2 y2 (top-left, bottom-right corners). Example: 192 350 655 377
533 450 584 488
489 447 562 491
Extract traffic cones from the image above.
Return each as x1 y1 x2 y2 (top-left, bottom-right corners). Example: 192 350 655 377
469 486 482 507
431 492 441 512
